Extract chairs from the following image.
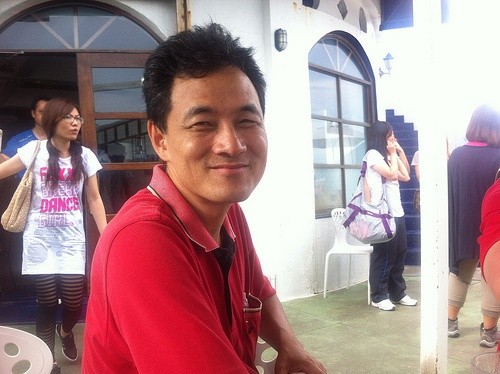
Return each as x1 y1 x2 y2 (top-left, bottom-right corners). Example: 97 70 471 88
323 207 374 305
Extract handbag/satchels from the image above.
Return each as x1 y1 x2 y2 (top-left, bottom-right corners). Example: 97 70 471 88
344 178 396 244
1 140 41 233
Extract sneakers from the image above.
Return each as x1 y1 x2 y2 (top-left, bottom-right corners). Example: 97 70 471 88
371 299 395 310
56 323 78 361
53 363 59 373
391 293 417 307
447 319 459 336
479 323 500 347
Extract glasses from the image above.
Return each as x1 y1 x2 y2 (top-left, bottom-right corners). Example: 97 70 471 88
63 113 85 125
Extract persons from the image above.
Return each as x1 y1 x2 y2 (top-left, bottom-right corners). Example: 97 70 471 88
412 139 421 215
79 22 329 373
475 166 500 356
359 119 418 312
446 103 500 346
0 99 67 315
0 99 109 374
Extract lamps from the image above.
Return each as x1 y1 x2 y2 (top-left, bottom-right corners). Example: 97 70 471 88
379 53 395 78
